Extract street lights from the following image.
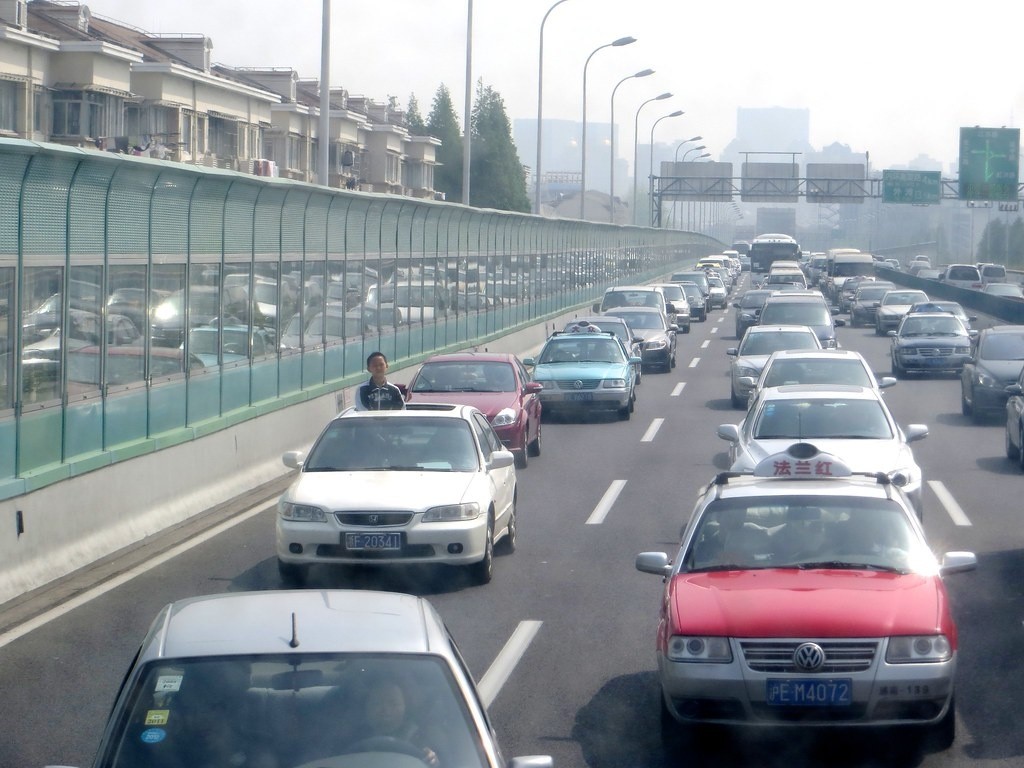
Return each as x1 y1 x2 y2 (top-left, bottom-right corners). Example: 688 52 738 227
650 109 686 226
610 67 655 224
677 137 713 227
580 35 638 219
635 92 674 223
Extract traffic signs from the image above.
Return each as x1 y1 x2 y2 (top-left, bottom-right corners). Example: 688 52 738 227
959 127 1020 203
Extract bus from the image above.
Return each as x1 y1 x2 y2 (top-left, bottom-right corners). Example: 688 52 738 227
732 241 750 254
747 233 803 289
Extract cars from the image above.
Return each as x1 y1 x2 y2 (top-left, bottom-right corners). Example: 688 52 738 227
563 280 709 381
276 407 517 583
716 384 929 524
396 355 544 470
741 257 750 269
803 249 1024 470
0 251 649 405
726 258 846 407
524 324 641 421
670 250 742 311
738 349 899 414
93 587 554 768
634 451 976 749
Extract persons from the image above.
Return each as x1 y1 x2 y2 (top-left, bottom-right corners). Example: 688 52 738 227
330 678 441 768
834 508 893 556
694 509 754 564
354 352 406 410
